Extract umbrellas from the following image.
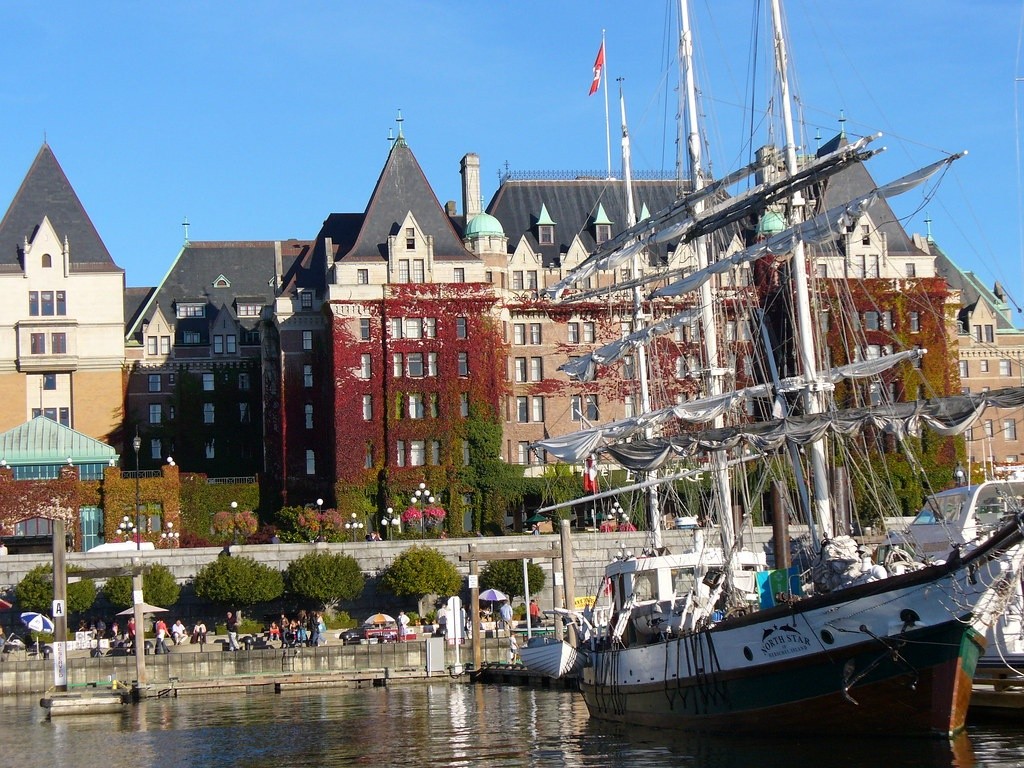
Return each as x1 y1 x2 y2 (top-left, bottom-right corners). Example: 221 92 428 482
365 613 395 629
523 513 549 522
116 603 170 616
478 589 507 601
20 612 54 660
584 513 602 521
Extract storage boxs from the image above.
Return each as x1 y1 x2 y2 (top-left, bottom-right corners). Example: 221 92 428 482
360 639 368 644
369 638 378 645
66 631 108 651
480 629 504 639
402 634 417 640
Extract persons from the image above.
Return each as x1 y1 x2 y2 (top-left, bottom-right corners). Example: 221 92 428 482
476 530 484 537
530 599 538 628
270 610 327 647
500 600 513 638
172 620 188 645
435 604 448 641
78 617 119 639
155 616 171 654
367 531 385 541
509 632 519 664
192 621 206 644
464 616 471 639
223 612 239 651
396 610 410 643
127 617 135 654
531 524 539 535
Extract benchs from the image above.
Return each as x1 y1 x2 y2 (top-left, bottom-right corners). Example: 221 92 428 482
253 630 309 650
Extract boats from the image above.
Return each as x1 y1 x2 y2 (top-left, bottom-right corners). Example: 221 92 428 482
874 480 1024 667
520 636 587 681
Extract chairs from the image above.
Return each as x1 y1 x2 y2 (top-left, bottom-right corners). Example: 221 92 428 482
214 639 230 651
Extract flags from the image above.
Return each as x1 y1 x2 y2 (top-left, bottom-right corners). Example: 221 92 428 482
589 42 605 96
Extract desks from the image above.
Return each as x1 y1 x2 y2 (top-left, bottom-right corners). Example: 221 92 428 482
509 627 556 642
365 629 399 643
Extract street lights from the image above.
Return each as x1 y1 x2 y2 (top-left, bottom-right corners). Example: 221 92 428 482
231 501 238 544
345 513 364 542
134 435 144 551
161 522 180 555
955 471 963 486
316 498 324 538
606 501 630 531
117 516 137 542
411 483 435 539
381 508 399 541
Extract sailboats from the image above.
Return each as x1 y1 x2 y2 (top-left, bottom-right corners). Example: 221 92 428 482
532 0 1023 731
